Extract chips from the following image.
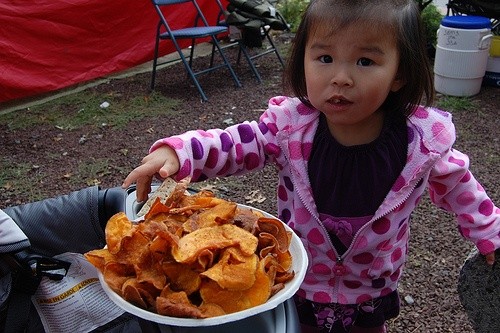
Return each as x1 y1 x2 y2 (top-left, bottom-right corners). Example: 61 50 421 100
84 181 295 320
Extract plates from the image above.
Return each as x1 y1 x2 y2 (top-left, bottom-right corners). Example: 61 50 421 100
98 203 309 326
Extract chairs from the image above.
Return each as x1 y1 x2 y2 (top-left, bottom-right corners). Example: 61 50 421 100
0 181 301 333
209 0 286 83
150 0 242 102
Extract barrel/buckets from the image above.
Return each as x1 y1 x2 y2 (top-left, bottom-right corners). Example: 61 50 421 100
433 15 493 97
489 35 500 57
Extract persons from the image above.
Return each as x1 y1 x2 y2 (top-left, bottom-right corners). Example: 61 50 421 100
121 0 500 333
